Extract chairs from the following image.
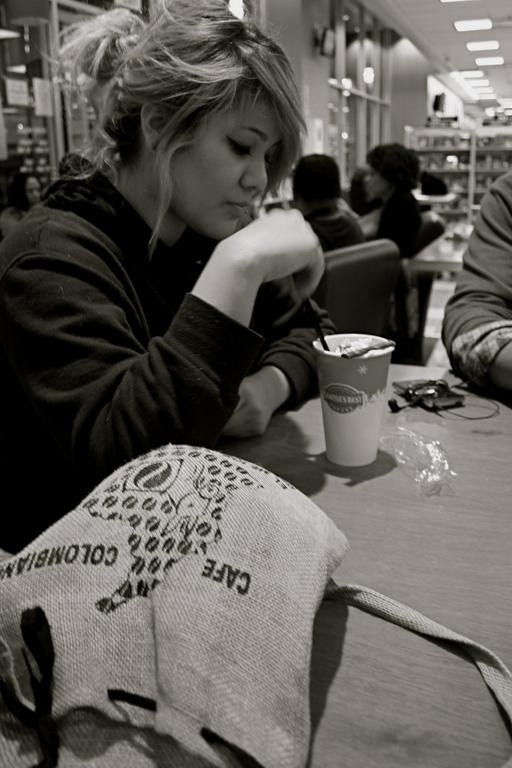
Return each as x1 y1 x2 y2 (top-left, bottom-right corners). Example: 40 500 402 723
315 200 448 367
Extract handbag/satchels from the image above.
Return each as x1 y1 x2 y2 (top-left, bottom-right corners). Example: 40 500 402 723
1 442 351 768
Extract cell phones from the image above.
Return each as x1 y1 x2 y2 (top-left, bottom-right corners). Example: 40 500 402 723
392 378 465 409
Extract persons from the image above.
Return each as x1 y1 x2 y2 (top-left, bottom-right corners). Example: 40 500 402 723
0 0 327 556
440 172 511 409
289 152 363 256
0 172 43 241
364 142 424 262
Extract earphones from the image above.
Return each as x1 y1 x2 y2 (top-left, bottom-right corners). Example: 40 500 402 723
388 399 400 413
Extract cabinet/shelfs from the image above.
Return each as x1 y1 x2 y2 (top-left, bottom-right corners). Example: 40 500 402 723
403 123 512 226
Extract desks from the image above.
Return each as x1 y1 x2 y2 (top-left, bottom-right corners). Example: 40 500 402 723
410 223 475 273
212 363 511 768
411 188 456 204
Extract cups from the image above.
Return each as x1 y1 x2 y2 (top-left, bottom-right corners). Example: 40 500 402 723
311 332 396 468
